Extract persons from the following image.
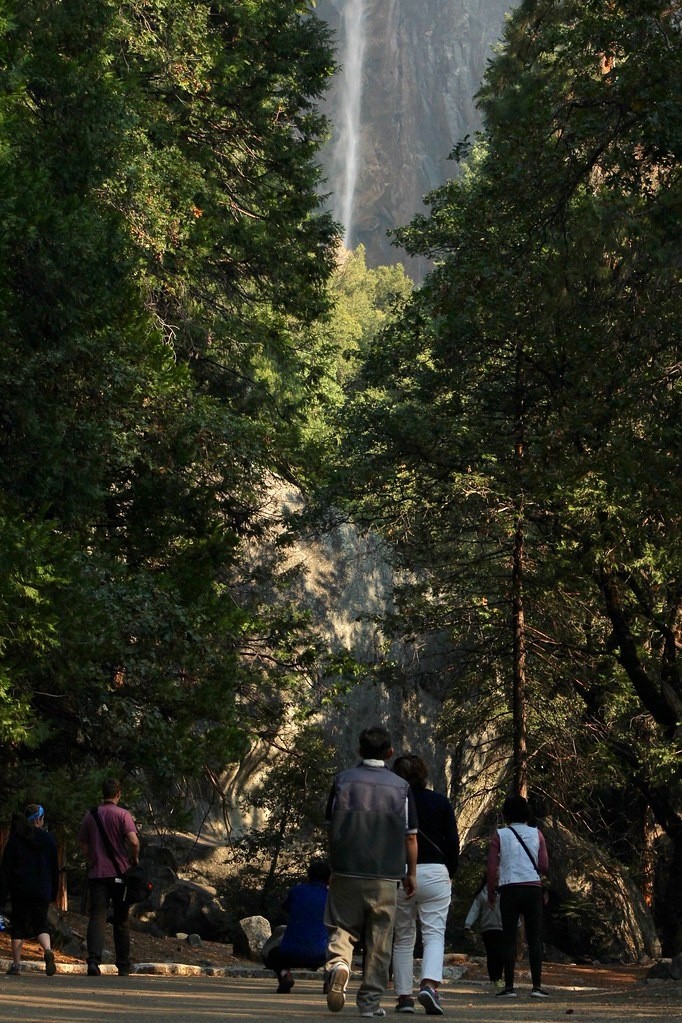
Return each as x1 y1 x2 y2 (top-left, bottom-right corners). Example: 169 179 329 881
77 777 140 976
487 794 550 997
462 863 522 988
0 804 57 976
260 858 331 994
323 725 419 1018
392 755 460 1015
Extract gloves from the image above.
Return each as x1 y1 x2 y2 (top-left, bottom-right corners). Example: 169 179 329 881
464 930 477 939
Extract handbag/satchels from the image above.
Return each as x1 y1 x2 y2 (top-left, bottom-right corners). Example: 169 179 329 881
109 865 152 907
539 872 551 886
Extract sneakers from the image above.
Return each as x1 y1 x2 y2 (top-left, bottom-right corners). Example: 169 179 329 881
530 988 549 997
418 986 443 1015
327 962 349 1012
395 998 414 1012
277 969 294 994
362 1008 386 1017
495 988 517 998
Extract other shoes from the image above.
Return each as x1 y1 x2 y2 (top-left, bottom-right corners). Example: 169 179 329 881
115 960 137 975
6 963 22 974
45 949 56 976
88 962 101 975
